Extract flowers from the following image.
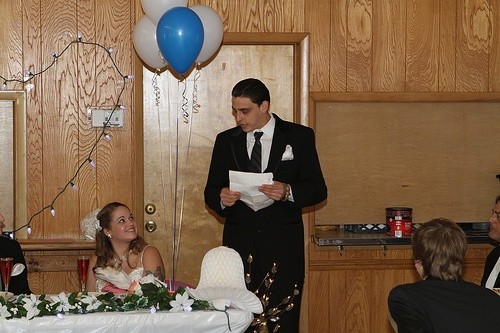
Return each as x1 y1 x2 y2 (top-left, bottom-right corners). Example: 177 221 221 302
0 267 232 331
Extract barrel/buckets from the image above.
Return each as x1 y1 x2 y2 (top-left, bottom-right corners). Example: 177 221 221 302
386 205 413 236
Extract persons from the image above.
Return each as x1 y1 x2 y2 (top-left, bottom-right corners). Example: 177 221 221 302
0 214 34 296
204 78 327 333
86 202 165 294
480 195 500 296
386 218 500 333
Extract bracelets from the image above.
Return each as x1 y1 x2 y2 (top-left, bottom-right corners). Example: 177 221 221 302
282 183 290 202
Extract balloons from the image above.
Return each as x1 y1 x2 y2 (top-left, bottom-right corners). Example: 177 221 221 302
156 6 204 77
132 15 168 71
188 5 224 65
141 0 188 25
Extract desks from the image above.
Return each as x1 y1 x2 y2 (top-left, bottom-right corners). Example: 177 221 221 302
0 293 254 333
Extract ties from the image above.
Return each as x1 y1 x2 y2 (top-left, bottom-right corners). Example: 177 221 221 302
493 271 500 288
251 131 263 173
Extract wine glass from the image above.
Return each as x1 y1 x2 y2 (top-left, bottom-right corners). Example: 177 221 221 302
76 255 90 293
0 257 14 303
